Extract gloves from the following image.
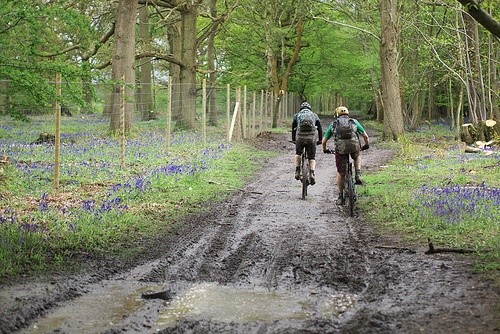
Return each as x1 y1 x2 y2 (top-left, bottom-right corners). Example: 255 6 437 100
323 148 331 153
361 144 369 150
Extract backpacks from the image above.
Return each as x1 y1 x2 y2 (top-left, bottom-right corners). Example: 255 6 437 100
299 109 316 132
333 117 353 139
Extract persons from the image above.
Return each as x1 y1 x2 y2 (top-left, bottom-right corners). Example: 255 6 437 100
291 102 323 186
321 106 370 205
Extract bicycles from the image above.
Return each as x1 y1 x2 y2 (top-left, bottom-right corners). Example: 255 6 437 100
289 140 322 200
323 146 369 217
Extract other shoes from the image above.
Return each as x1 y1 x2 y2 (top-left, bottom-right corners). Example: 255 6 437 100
336 192 343 205
355 168 362 184
309 168 316 186
294 165 301 180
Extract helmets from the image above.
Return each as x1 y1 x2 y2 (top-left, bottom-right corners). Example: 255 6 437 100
334 106 349 117
300 102 312 110
